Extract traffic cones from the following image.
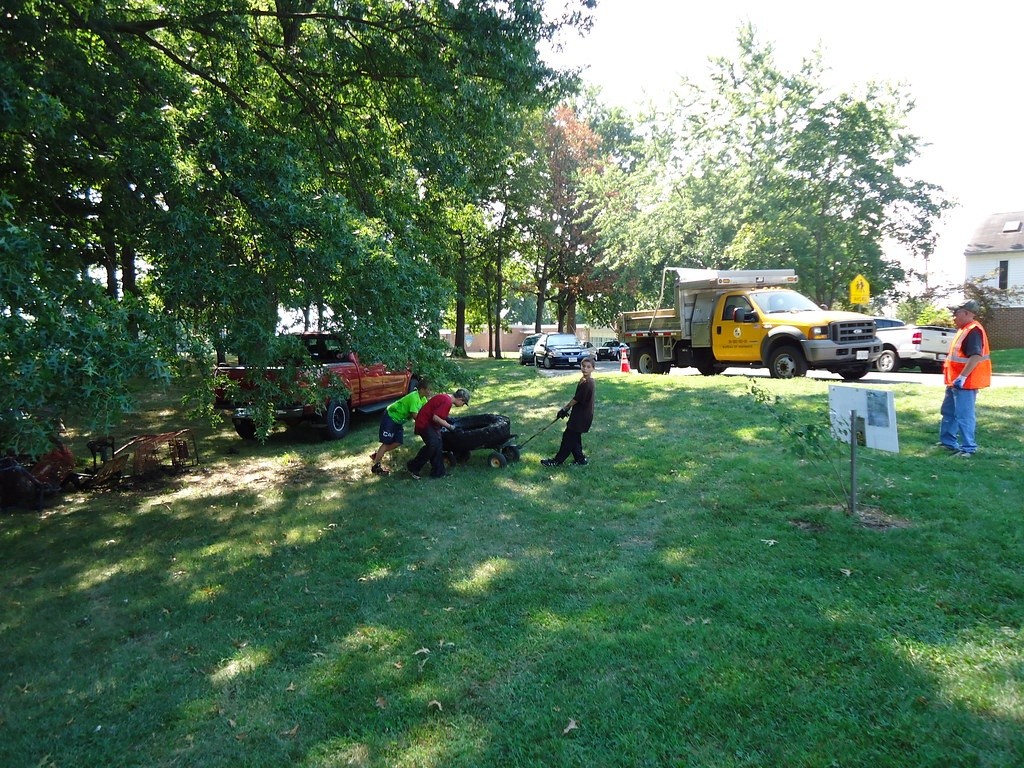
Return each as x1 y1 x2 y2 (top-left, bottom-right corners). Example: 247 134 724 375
621 349 630 372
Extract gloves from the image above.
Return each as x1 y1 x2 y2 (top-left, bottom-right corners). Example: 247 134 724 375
447 417 455 425
449 424 462 434
953 375 967 390
557 406 570 420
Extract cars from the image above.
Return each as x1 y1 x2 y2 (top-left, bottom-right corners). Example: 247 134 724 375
533 332 590 369
580 341 597 360
518 335 540 365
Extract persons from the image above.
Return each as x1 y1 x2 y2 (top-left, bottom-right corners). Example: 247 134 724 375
936 299 992 454
405 388 470 480
540 357 596 468
370 380 431 476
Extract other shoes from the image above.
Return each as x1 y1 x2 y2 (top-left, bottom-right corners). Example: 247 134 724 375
372 465 389 476
954 451 974 459
434 473 453 478
370 453 382 461
405 462 421 479
931 444 951 452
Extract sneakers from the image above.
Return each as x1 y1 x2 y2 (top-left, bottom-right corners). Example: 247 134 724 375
541 458 562 466
569 458 587 466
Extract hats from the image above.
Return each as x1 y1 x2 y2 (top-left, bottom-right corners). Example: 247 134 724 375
457 389 471 407
947 299 979 314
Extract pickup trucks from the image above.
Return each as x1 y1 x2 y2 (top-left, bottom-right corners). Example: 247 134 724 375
599 341 630 361
872 317 957 373
623 267 882 379
212 332 421 440
